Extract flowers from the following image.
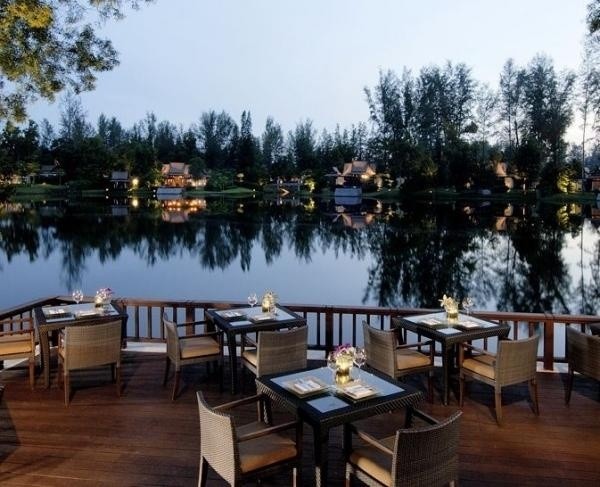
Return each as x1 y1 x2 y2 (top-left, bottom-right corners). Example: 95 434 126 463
327 343 368 368
438 293 458 310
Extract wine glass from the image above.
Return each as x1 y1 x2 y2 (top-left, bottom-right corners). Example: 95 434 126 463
461 296 476 319
353 346 368 382
327 350 340 394
248 293 260 314
72 289 84 312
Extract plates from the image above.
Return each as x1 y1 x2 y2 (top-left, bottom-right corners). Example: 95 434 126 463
416 314 445 331
40 306 72 319
74 308 104 320
331 382 383 402
451 320 486 330
248 312 276 323
213 308 246 321
280 375 331 400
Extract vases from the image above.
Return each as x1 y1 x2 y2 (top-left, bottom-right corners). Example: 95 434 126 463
447 309 457 327
333 366 351 386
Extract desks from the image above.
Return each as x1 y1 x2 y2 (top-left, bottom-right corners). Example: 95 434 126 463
392 310 511 406
204 304 307 394
33 302 128 390
255 364 425 486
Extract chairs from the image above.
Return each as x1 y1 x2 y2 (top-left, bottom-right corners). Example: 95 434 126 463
196 390 297 487
0 317 35 392
240 325 308 421
565 325 600 405
58 320 122 407
362 320 435 414
162 317 224 400
459 334 540 426
341 408 463 487
